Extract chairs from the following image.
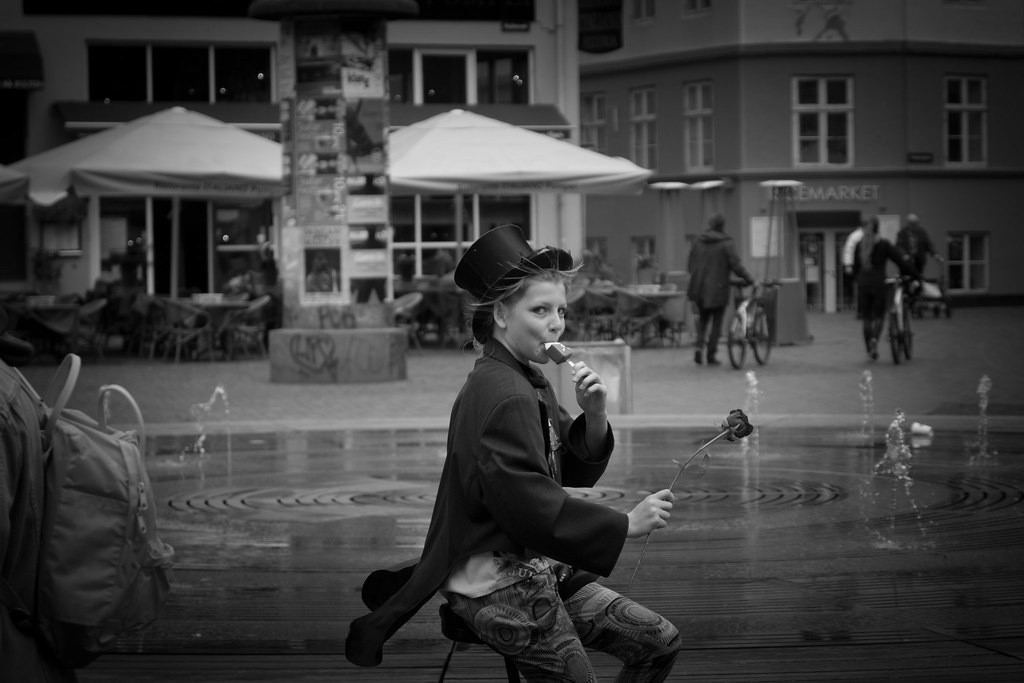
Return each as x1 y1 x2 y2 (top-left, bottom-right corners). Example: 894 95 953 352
4 270 696 363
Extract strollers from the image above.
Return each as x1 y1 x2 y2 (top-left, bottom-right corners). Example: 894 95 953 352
912 258 953 321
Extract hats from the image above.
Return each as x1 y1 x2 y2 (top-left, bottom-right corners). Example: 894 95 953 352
455 225 573 346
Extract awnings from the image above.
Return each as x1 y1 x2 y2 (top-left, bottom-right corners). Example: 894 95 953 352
1 101 656 323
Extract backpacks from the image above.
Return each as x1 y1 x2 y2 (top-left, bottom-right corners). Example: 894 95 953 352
12 356 176 664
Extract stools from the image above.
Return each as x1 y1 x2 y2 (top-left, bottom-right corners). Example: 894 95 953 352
438 603 525 683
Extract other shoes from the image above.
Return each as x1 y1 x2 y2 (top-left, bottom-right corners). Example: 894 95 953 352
869 337 879 360
707 353 722 366
693 347 703 366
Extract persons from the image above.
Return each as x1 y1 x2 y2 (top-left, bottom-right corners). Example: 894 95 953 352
889 215 947 319
0 344 81 682
346 224 687 683
77 232 292 357
685 212 758 365
393 232 676 355
844 213 914 359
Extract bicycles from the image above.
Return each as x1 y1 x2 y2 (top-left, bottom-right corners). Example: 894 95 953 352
884 274 913 367
727 276 783 367
